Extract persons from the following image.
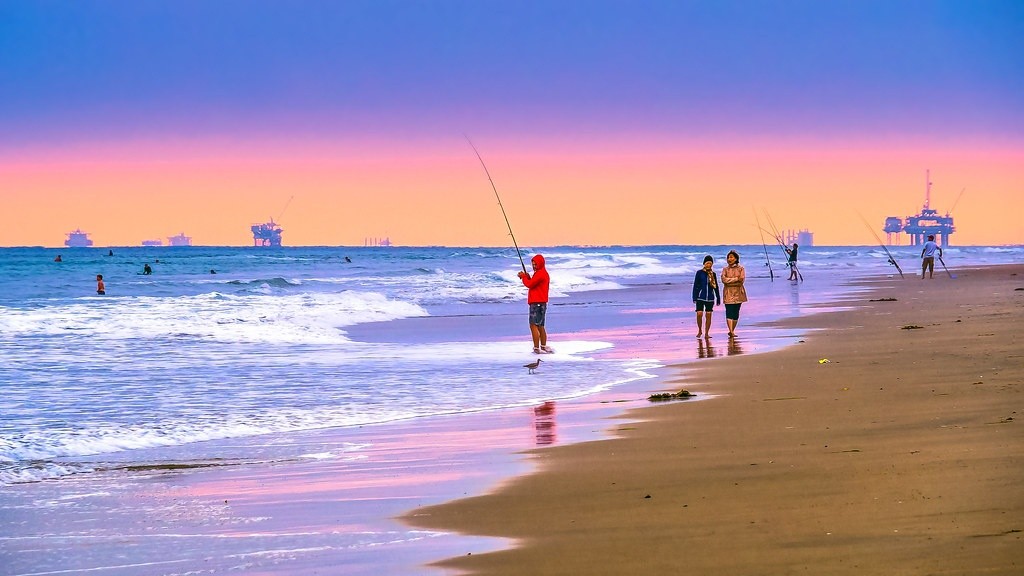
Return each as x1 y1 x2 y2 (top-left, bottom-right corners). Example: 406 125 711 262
692 255 720 338
55 255 62 262
921 235 942 279
97 274 106 296
345 257 351 262
721 251 748 337
142 264 151 275
109 249 113 256
210 269 216 274
787 243 799 280
517 254 550 354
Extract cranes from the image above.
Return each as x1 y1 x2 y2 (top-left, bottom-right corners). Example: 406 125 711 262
924 168 966 218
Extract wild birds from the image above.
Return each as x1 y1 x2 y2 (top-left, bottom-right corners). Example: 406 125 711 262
523 359 544 374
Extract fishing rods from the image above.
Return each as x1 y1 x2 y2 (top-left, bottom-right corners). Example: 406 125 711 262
752 208 804 282
865 221 905 275
753 211 774 282
461 129 528 275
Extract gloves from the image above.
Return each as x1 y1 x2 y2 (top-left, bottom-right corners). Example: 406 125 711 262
526 273 530 278
517 271 524 279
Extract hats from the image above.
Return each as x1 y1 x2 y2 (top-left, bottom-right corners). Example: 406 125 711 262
927 234 934 238
703 256 712 265
